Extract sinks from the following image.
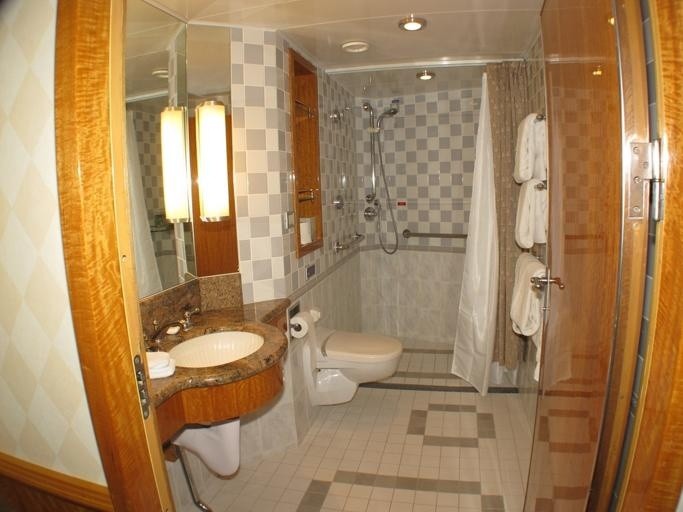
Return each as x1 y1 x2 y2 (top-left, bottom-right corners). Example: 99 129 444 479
170 331 264 368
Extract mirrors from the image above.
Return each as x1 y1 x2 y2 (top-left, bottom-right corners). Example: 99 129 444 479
126 2 197 304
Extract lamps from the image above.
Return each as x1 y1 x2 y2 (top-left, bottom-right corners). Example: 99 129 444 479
415 71 436 81
195 98 229 224
397 17 427 33
159 106 194 226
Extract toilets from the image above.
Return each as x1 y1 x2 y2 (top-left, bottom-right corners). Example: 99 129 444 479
302 328 403 406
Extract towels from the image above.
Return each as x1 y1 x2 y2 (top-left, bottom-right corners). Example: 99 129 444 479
509 111 549 382
145 351 175 379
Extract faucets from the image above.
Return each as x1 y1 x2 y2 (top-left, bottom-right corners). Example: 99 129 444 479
152 322 188 341
